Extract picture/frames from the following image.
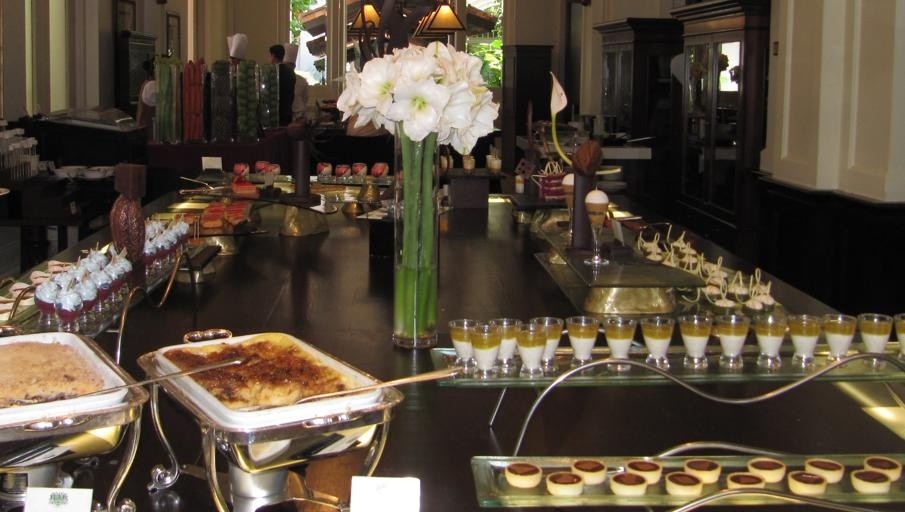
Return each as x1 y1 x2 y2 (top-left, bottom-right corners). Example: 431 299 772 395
111 0 137 47
164 10 183 61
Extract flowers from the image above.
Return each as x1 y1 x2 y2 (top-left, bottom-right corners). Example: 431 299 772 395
329 37 507 355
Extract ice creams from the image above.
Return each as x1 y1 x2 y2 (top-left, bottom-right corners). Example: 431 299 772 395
584 188 609 226
562 173 574 209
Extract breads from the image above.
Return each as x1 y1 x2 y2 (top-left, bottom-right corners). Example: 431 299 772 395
201 201 251 227
231 180 256 195
174 213 201 225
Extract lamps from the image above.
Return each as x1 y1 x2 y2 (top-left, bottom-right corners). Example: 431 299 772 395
348 0 467 39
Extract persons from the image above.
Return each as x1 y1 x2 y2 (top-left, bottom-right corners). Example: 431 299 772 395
229 57 244 67
285 62 310 124
266 44 294 128
136 54 172 122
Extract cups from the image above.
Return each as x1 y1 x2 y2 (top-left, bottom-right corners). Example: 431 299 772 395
450 311 905 382
638 238 775 314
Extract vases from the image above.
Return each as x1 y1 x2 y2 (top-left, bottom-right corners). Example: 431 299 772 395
387 113 450 352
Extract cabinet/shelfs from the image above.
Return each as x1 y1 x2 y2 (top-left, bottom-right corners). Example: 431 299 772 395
666 1 772 255
483 42 558 178
588 14 684 140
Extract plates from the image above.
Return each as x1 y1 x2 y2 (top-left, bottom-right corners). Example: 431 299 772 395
1 186 12 197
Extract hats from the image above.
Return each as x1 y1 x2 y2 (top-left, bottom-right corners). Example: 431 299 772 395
281 43 299 64
226 32 250 63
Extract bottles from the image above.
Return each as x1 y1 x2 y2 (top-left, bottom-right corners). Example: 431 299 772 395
0 119 38 183
151 61 279 143
441 153 502 177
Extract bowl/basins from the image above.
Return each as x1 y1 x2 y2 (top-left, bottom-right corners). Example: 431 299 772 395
624 458 662 484
505 463 542 489
724 471 766 490
605 470 647 498
746 459 786 481
789 471 826 496
571 460 607 486
53 165 115 180
544 471 583 496
860 456 901 483
805 458 845 482
850 471 889 495
665 472 701 498
685 457 719 484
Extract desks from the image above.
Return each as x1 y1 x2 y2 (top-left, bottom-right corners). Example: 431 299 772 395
139 123 292 193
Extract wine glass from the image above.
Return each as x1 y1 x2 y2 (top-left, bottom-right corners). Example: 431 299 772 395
32 249 133 331
145 217 193 275
582 203 612 268
558 182 573 247
